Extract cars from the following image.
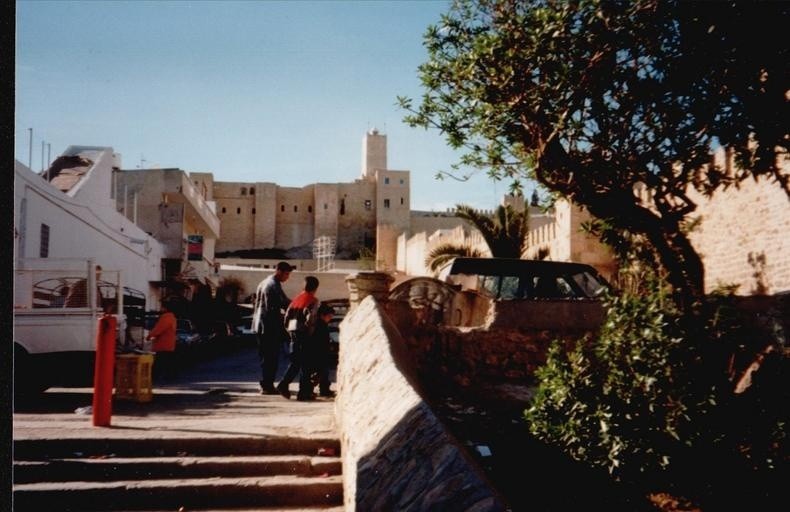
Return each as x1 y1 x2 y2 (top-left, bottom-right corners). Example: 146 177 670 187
147 303 353 370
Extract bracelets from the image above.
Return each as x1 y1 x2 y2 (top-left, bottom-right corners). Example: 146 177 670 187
133 342 137 345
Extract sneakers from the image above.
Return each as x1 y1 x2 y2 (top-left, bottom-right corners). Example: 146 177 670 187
296 390 316 401
276 385 292 399
319 389 335 396
261 384 276 394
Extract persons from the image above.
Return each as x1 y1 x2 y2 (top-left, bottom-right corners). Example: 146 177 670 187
313 304 338 398
146 299 181 386
106 290 141 351
62 264 104 307
275 276 319 401
250 262 293 394
48 288 71 308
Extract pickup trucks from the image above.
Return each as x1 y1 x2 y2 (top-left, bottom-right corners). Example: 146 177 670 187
11 255 127 413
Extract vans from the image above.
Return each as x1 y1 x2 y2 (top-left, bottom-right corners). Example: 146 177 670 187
432 258 623 300
